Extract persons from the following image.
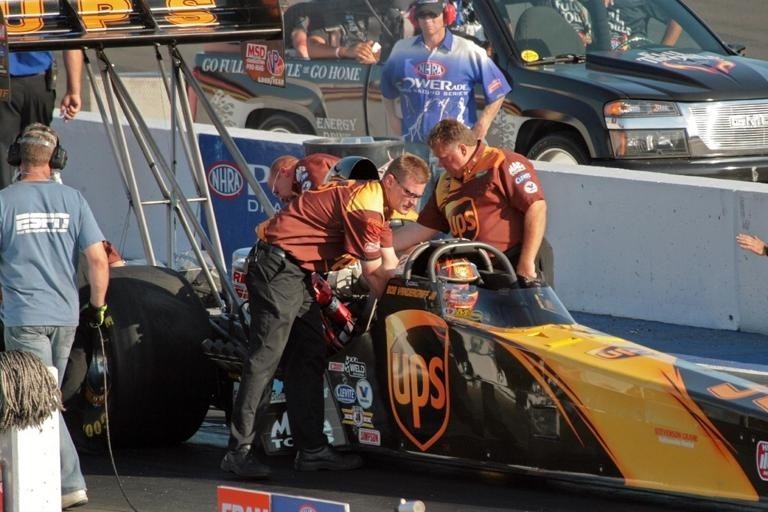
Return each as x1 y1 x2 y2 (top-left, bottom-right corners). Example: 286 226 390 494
1 31 82 184
267 153 351 203
0 123 111 512
380 1 514 149
307 0 384 65
554 0 681 52
219 155 431 481
281 0 313 62
737 234 766 256
391 119 554 285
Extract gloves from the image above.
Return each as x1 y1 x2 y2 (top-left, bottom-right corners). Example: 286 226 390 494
86 300 107 330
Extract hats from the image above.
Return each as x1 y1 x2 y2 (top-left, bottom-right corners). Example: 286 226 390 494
414 0 444 17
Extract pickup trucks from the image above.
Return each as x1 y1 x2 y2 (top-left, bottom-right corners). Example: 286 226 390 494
186 1 768 183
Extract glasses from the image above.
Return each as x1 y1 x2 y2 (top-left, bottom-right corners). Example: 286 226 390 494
271 170 280 196
390 172 423 199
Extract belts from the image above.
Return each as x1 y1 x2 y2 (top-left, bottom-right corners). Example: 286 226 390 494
256 240 287 259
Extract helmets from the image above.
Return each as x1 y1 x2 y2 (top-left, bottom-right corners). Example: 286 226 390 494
434 254 485 310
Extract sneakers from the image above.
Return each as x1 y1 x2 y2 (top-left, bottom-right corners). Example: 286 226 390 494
219 443 274 480
293 435 365 472
61 487 89 509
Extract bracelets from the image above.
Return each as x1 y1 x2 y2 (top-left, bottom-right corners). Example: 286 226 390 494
335 47 340 58
